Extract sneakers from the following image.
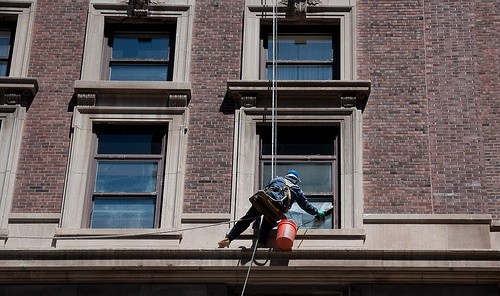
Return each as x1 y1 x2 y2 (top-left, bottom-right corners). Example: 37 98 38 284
216 237 230 247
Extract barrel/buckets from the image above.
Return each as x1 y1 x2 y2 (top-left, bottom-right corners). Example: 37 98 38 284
276 219 297 249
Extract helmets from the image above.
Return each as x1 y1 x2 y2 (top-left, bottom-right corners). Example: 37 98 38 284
286 170 301 181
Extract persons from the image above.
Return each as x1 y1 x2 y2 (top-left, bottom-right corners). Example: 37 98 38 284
218 170 326 251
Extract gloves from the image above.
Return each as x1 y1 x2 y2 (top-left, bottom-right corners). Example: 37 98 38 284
317 209 327 217
